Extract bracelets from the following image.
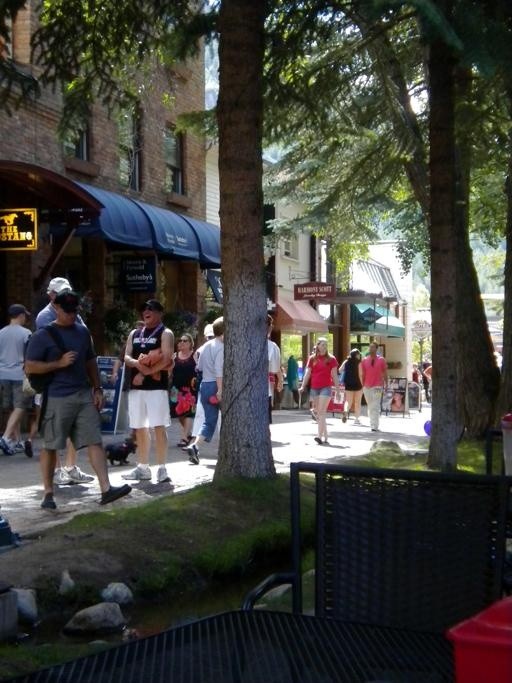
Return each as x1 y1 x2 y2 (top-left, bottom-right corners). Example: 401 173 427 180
93 386 103 392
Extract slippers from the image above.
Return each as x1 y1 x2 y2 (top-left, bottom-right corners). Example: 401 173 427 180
314 437 323 444
323 441 329 443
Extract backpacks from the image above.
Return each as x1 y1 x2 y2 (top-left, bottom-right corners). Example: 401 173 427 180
22 326 71 392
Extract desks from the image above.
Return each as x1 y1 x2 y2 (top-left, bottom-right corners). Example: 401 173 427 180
1 609 455 681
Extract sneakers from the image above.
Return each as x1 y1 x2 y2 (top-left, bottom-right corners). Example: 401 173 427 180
371 428 377 432
99 484 133 506
187 443 199 465
41 491 56 512
157 467 172 483
121 466 152 481
309 408 318 422
354 419 360 424
64 465 95 483
52 468 74 485
25 438 34 459
0 435 16 455
341 410 349 423
11 440 26 453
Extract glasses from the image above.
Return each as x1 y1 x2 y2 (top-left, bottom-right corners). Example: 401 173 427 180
140 306 155 312
177 339 189 342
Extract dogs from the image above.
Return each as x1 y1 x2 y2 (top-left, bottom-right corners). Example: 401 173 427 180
104 440 138 465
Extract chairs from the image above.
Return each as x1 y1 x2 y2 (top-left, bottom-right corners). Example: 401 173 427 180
242 462 511 634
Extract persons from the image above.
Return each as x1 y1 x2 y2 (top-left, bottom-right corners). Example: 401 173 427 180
361 341 389 432
110 299 224 484
392 393 404 410
424 366 432 391
25 393 42 457
0 304 32 454
339 349 363 425
270 371 284 392
36 277 95 485
412 365 419 384
299 337 341 446
23 289 131 510
267 314 281 438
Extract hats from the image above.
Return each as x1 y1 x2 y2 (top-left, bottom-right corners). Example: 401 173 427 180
8 303 32 317
139 299 164 311
46 276 73 295
54 293 82 314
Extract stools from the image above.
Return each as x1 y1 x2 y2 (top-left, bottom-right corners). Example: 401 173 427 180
485 427 506 476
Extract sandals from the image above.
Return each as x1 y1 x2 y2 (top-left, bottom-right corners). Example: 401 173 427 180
176 437 189 447
